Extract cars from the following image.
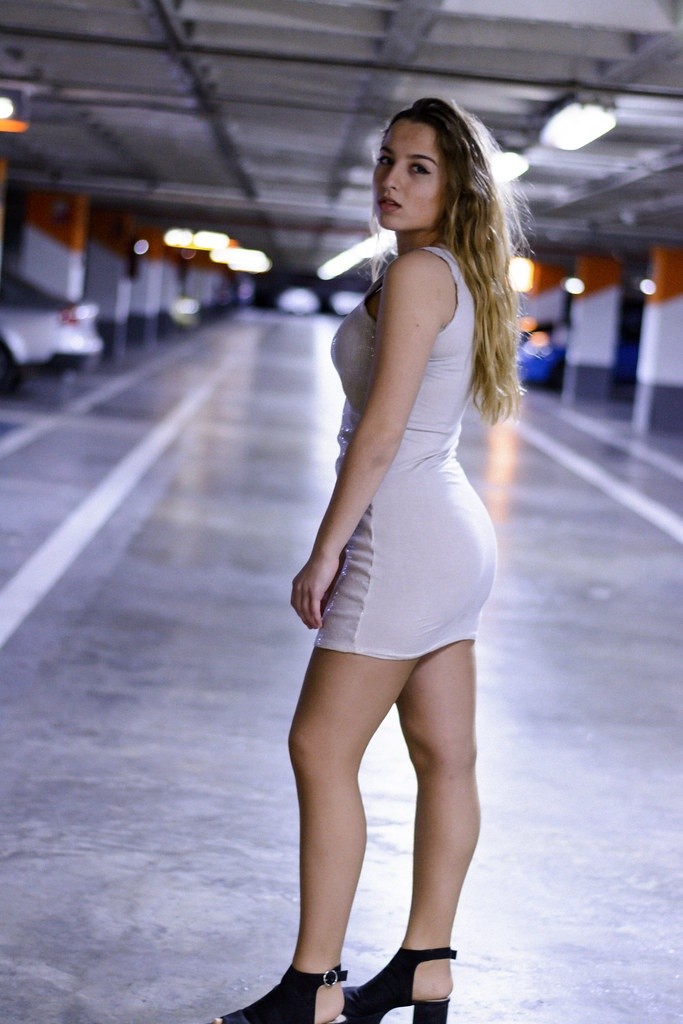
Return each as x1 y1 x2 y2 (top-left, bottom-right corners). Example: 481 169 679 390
0 265 102 399
515 319 636 389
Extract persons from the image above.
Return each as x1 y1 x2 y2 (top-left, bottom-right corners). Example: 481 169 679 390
210 97 522 1024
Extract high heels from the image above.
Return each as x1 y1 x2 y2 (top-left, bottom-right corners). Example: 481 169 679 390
342 947 457 1024
209 965 348 1024
